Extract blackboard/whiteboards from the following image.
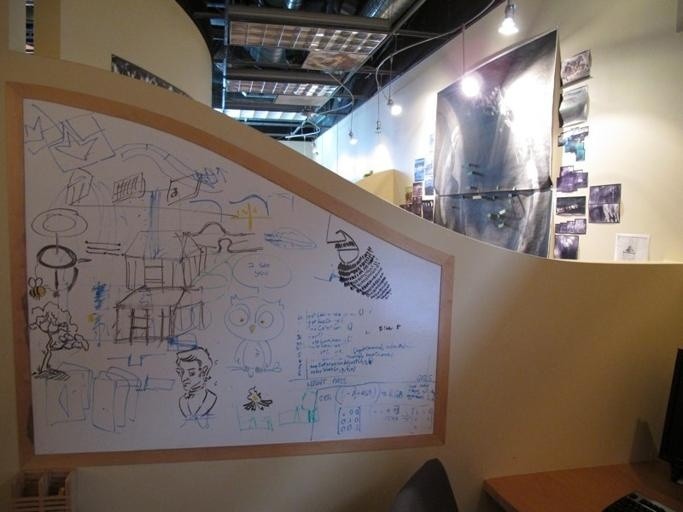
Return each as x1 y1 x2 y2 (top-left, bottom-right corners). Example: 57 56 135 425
3 82 455 473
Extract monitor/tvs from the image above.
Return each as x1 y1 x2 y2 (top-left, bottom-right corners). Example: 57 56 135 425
658 349 682 485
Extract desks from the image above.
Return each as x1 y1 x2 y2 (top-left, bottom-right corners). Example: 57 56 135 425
483 460 683 512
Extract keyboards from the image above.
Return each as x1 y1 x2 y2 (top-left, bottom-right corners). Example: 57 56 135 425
602 492 676 512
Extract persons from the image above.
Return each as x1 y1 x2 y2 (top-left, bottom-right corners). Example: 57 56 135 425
437 94 541 254
